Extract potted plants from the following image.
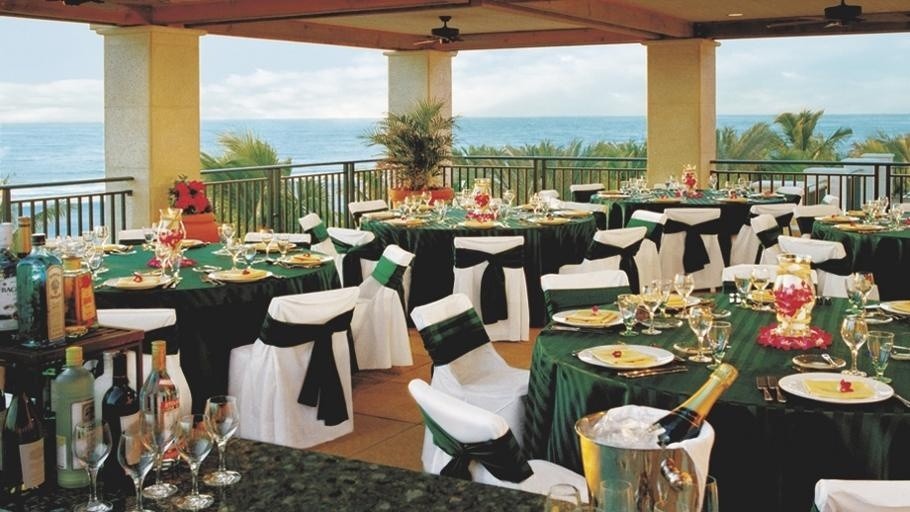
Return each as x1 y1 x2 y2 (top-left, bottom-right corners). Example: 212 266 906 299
356 95 463 206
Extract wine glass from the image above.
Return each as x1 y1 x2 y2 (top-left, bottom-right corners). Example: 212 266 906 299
79 206 291 283
733 264 874 317
688 305 730 370
866 331 895 384
66 397 244 512
617 273 695 335
841 315 868 377
616 167 905 232
388 180 555 223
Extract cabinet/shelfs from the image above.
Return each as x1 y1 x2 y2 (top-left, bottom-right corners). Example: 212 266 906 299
2 319 144 420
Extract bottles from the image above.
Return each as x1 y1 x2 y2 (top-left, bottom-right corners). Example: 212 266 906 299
0 337 179 492
648 362 740 448
773 252 818 338
0 216 97 348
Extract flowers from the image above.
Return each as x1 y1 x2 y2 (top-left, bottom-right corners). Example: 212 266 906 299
169 179 211 215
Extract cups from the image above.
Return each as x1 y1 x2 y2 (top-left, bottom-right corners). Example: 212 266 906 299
542 474 718 511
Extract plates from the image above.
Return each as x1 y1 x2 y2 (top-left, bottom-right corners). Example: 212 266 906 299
273 253 339 267
778 373 895 406
793 354 846 371
879 300 910 315
641 308 730 329
577 342 673 372
549 307 624 328
674 341 714 356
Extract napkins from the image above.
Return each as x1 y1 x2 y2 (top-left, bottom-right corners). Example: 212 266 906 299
115 275 156 288
288 253 324 263
222 268 265 278
804 378 878 398
589 344 657 368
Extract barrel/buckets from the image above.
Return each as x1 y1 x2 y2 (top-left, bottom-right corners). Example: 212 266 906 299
575 404 715 512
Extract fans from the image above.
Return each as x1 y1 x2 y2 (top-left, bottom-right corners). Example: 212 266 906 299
764 0 910 37
380 15 498 54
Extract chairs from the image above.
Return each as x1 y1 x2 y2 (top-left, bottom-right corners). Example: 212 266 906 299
299 182 910 342
228 286 360 449
346 245 416 371
814 479 908 511
408 378 588 505
119 229 147 245
410 292 530 470
244 232 311 250
94 308 191 416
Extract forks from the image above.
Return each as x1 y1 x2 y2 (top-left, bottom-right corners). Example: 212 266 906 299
755 374 786 403
